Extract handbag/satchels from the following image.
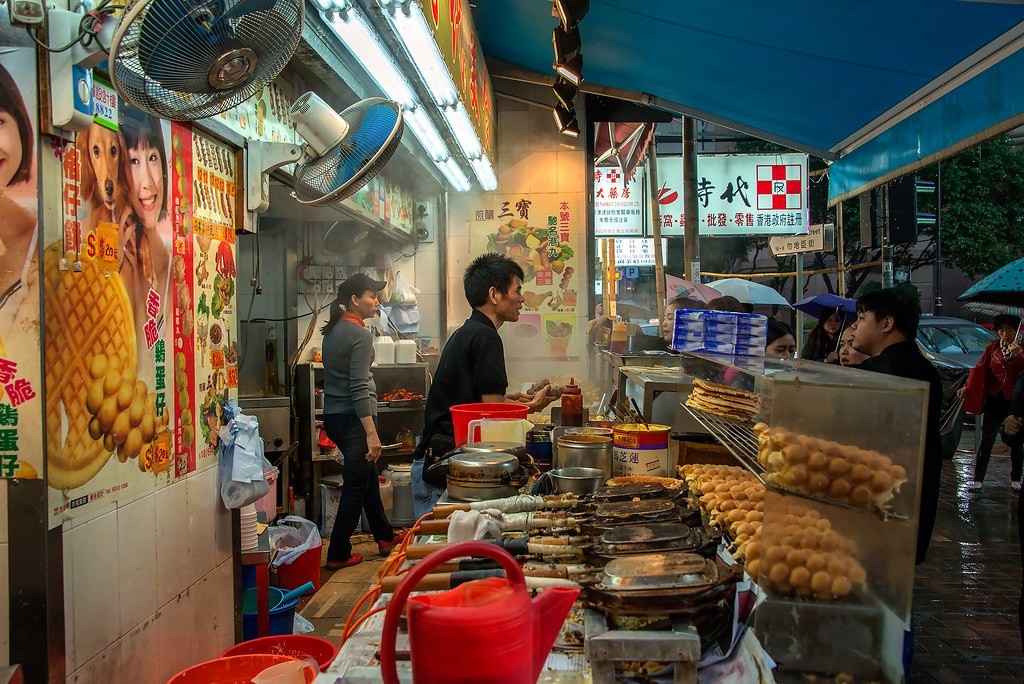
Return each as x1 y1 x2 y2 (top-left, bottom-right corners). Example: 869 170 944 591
216 438 271 509
421 434 455 490
962 340 998 416
271 514 322 567
391 271 418 306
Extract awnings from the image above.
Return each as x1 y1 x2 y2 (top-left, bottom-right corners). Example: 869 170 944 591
469 0 1024 296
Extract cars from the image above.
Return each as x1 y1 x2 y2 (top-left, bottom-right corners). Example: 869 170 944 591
915 311 1000 399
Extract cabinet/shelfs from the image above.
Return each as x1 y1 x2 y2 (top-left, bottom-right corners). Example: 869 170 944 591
294 363 430 534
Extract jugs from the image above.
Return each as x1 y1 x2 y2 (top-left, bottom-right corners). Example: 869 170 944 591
467 418 534 450
381 542 581 684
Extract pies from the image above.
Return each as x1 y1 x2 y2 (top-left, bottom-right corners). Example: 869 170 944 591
686 378 761 421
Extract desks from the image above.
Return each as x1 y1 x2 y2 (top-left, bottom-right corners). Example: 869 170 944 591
241 511 271 638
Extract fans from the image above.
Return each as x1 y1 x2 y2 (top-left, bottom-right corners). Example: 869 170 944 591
247 91 405 296
321 219 374 256
47 0 303 272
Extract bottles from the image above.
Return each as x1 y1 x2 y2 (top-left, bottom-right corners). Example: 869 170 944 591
362 475 393 533
627 335 634 352
561 377 583 428
612 316 627 353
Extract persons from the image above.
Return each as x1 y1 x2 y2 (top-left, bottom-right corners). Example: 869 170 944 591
323 273 408 567
706 296 754 313
841 328 870 367
0 62 39 344
662 299 705 343
801 309 845 362
955 316 1024 491
411 253 561 521
768 321 796 357
112 106 174 348
845 287 943 564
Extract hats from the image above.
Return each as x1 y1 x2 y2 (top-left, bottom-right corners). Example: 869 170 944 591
337 272 388 302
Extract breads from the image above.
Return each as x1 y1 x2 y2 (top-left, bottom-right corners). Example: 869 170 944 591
40 237 141 490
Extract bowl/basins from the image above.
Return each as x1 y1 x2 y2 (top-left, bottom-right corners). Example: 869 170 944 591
240 502 258 550
551 467 606 495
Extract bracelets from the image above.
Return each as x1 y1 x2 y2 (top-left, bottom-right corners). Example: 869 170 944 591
823 358 828 362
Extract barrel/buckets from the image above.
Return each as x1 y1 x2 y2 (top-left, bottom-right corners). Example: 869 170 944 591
166 635 338 684
243 589 300 641
241 564 256 591
272 540 325 595
382 464 416 525
449 404 530 448
612 424 672 479
556 427 613 482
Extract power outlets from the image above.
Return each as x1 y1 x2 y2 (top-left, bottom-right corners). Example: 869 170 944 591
308 265 378 295
415 201 430 214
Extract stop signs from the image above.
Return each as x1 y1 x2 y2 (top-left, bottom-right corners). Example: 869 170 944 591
625 267 639 280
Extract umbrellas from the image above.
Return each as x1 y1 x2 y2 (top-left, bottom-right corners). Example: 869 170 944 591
706 277 795 309
959 256 1024 343
795 293 858 353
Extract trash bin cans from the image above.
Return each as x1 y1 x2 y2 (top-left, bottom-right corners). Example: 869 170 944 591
275 536 325 595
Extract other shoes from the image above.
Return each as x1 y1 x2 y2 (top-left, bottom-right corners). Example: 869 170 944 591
325 553 363 569
969 480 983 492
378 529 409 556
1011 480 1022 494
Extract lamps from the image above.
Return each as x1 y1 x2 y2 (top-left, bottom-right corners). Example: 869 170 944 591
563 117 582 138
552 21 582 64
553 105 577 133
320 4 421 112
380 4 460 109
401 103 451 163
432 158 473 193
556 0 589 32
557 53 585 86
553 76 578 112
466 154 498 191
438 103 483 159
